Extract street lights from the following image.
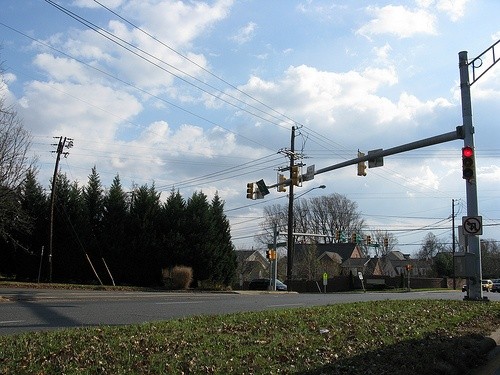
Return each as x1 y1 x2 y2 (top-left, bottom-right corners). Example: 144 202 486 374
286 185 326 291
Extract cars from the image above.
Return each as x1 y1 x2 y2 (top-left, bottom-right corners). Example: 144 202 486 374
246 278 287 290
462 285 468 293
482 279 500 294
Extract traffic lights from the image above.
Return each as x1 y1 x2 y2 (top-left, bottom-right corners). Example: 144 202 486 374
461 147 475 179
246 184 254 199
336 230 340 240
406 265 414 272
291 166 300 186
277 173 287 192
358 152 367 176
352 233 357 243
266 250 275 260
366 236 371 246
384 239 389 247
